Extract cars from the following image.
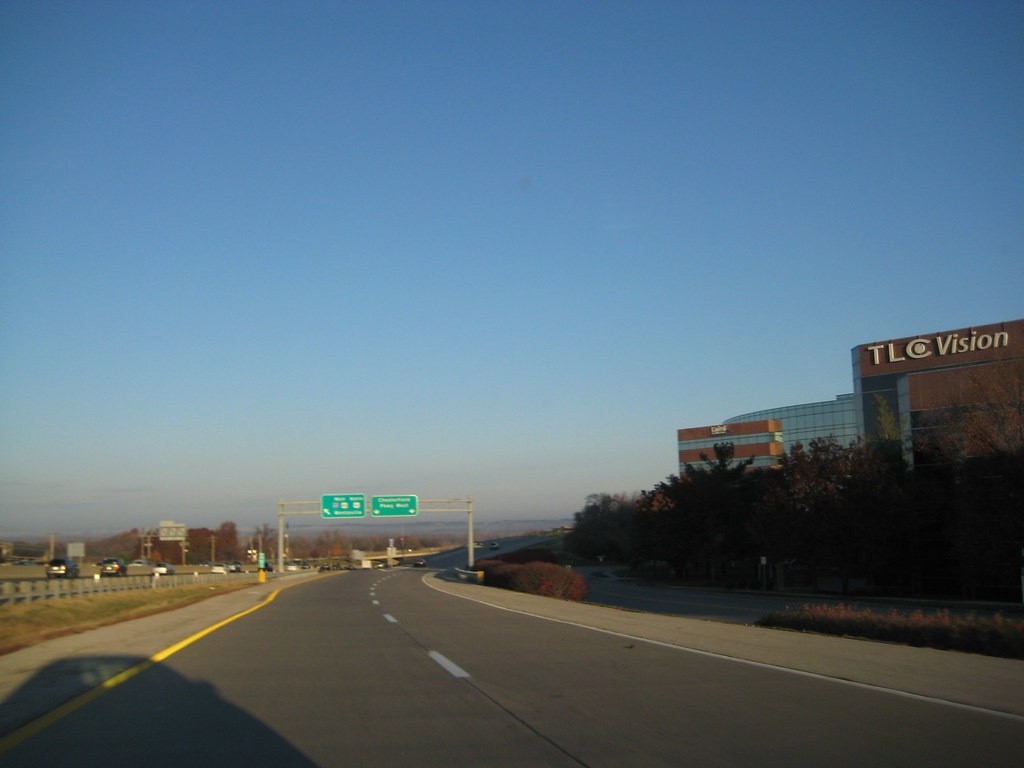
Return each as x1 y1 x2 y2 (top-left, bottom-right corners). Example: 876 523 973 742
264 562 273 571
210 562 240 575
100 557 128 577
414 559 426 568
46 559 81 578
489 542 500 550
152 563 174 576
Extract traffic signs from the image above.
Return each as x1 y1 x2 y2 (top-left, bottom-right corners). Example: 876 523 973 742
370 496 419 517
319 494 366 519
